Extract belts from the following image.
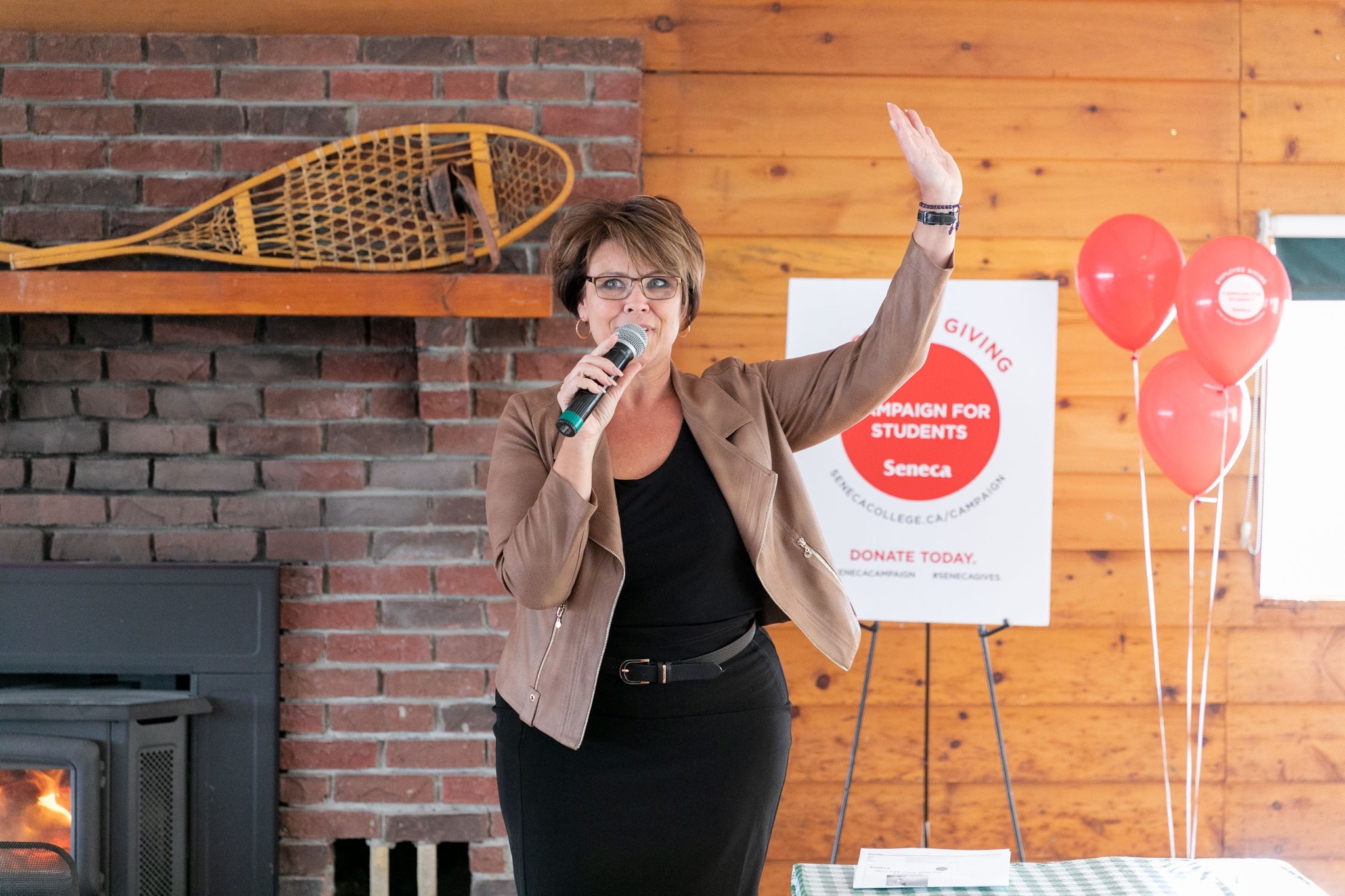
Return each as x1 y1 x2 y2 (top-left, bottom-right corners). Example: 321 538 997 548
599 618 758 685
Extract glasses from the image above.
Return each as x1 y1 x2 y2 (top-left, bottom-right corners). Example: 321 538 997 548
583 276 682 300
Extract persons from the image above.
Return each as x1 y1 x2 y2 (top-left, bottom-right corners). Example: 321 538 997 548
486 102 963 896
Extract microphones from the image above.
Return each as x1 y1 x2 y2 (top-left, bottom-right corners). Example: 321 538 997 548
556 323 648 438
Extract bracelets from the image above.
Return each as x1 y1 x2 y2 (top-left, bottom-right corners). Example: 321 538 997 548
917 202 960 235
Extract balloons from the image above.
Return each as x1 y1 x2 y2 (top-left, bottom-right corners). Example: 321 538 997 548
1138 350 1252 496
1075 214 1185 352
1175 236 1292 388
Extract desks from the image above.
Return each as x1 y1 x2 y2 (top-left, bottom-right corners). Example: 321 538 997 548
792 858 1331 896
